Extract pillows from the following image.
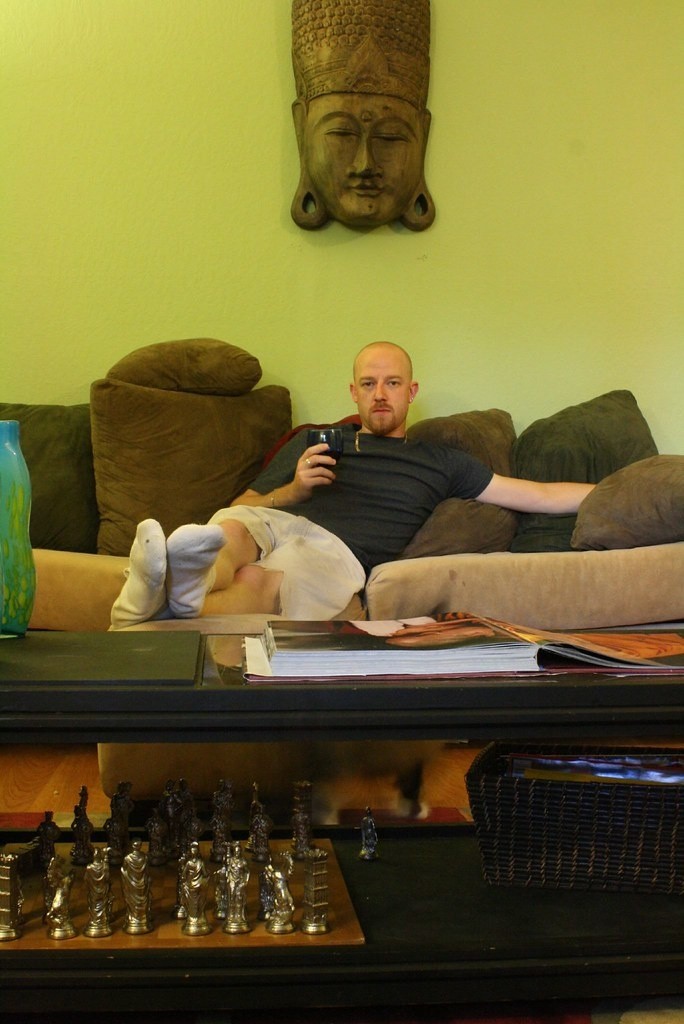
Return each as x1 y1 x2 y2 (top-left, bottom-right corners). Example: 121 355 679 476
397 407 517 559
106 338 263 396
510 390 659 551
570 454 684 551
91 379 291 556
0 401 99 554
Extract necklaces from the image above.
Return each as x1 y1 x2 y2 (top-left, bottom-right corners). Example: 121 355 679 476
355 431 407 452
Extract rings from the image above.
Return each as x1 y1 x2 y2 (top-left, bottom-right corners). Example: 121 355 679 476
306 458 310 464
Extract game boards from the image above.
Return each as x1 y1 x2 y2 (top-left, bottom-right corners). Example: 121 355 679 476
0 838 367 951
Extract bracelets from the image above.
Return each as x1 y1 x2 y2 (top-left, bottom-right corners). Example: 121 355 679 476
271 490 275 506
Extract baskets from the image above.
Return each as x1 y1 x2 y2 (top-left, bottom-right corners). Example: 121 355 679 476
464 738 683 896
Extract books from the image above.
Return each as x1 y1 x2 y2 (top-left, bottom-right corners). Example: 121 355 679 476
263 610 684 677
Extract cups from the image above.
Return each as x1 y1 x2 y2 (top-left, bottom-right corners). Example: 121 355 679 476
309 430 343 494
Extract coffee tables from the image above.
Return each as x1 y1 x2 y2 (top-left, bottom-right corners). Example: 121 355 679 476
0 623 684 1024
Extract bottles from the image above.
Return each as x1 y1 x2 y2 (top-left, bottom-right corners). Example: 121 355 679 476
0 420 34 637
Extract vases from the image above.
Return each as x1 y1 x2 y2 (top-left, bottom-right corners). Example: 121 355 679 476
0 420 38 638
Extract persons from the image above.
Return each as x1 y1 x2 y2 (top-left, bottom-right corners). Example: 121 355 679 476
289 0 436 231
110 341 598 633
37 774 381 936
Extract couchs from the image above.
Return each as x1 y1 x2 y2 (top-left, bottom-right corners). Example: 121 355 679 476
0 337 684 805
